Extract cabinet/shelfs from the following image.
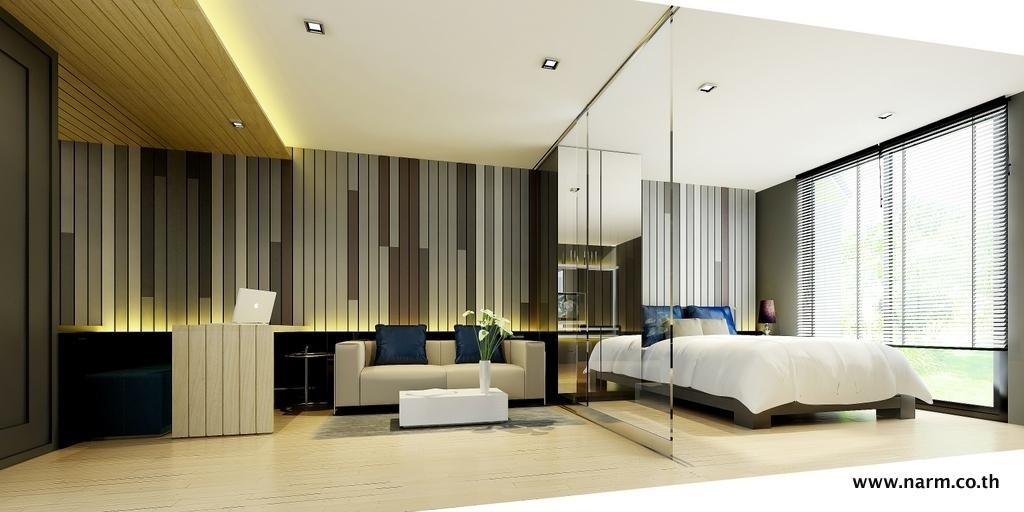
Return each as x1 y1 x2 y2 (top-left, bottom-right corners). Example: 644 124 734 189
165 321 276 439
395 386 509 430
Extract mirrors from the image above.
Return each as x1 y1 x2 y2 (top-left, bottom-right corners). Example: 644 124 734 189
550 144 644 401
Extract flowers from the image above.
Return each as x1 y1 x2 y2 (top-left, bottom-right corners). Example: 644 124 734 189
459 307 514 361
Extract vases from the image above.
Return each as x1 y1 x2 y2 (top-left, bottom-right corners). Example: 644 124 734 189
478 358 492 392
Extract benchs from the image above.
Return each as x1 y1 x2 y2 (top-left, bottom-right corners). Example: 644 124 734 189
85 363 170 440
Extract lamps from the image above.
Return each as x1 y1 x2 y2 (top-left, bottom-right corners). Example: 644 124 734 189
757 299 776 335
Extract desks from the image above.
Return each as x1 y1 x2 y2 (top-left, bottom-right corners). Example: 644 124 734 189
281 345 333 412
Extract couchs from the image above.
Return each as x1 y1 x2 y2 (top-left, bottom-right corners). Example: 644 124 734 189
330 338 547 416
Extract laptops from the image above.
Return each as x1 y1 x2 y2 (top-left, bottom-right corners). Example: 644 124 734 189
200 288 277 325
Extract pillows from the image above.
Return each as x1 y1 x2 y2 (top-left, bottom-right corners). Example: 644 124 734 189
372 322 430 366
695 316 730 335
639 305 684 347
685 304 738 336
452 323 506 364
663 317 704 339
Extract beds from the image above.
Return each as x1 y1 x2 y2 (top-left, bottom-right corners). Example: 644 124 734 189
641 328 934 429
582 333 643 394
